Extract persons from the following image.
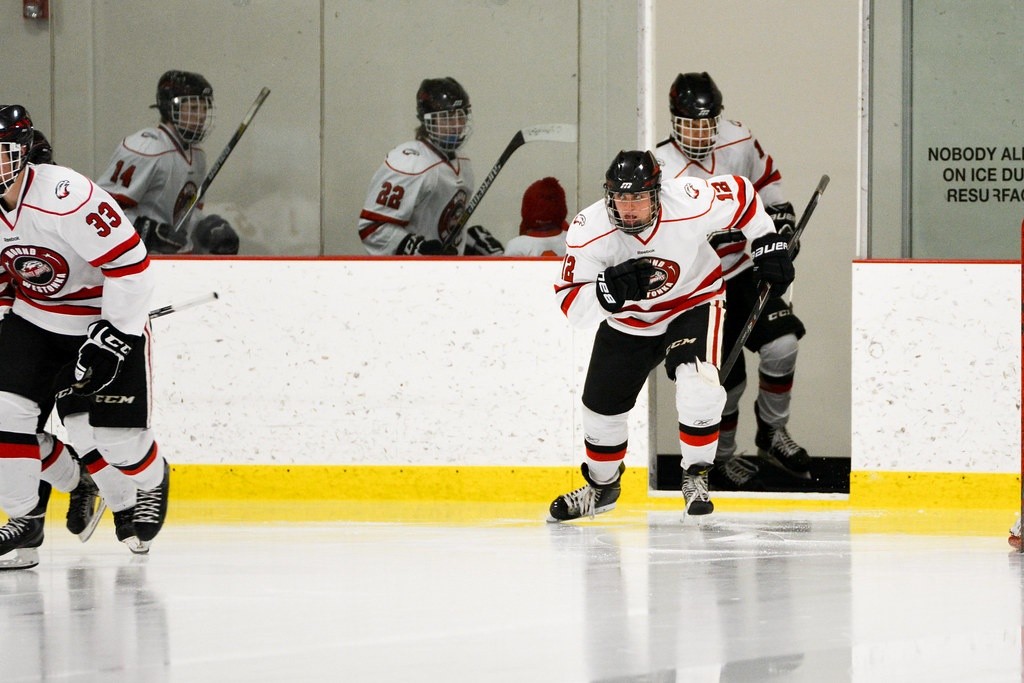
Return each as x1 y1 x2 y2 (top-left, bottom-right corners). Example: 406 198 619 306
546 149 795 527
503 177 571 257
0 103 170 572
357 77 504 256
28 129 151 554
651 72 811 493
94 69 240 255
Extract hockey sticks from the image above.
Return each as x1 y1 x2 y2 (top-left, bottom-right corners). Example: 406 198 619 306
148 292 219 320
718 173 831 387
442 121 580 254
172 85 272 234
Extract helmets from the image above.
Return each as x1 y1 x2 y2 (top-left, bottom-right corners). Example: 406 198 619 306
415 77 473 153
28 129 51 164
602 149 662 235
155 70 218 144
0 103 34 198
669 72 723 163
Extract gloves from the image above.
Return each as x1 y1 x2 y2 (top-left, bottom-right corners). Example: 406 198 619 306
71 319 142 396
764 202 801 261
596 258 656 313
395 234 459 257
466 225 504 256
133 214 187 254
751 232 796 300
195 213 240 254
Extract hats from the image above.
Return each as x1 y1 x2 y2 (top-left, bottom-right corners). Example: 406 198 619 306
520 177 568 232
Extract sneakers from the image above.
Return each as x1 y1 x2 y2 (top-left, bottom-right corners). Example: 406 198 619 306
712 450 769 491
681 460 714 526
113 507 148 553
130 456 170 546
57 443 107 543
546 460 625 523
754 400 813 480
0 478 51 571
